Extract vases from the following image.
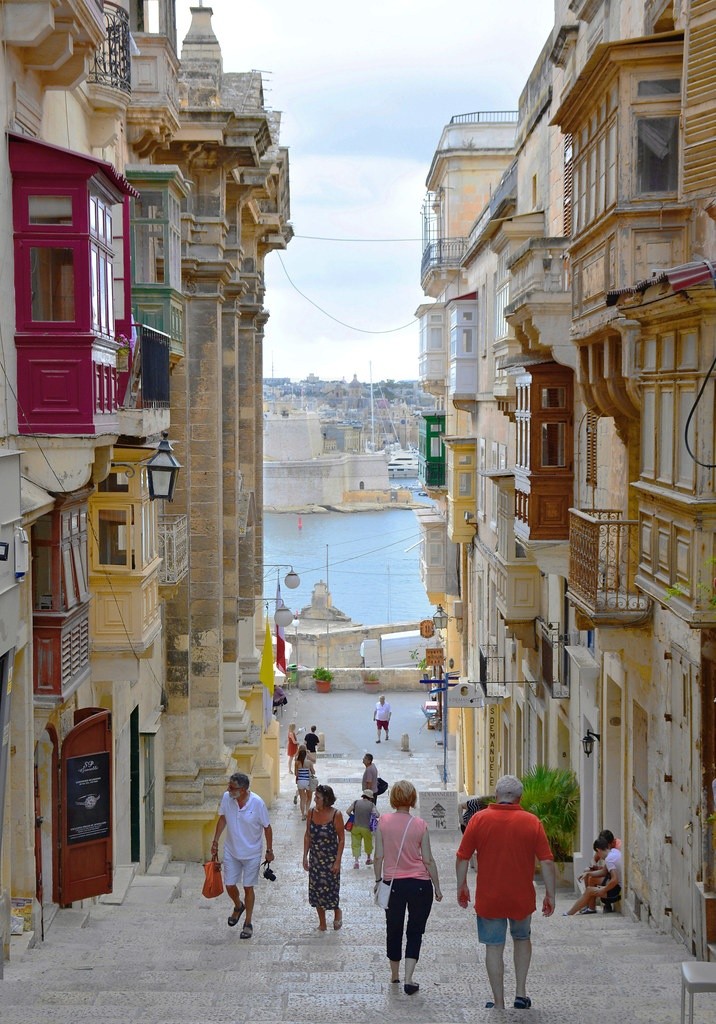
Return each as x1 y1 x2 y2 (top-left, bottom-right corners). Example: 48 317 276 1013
114 352 129 371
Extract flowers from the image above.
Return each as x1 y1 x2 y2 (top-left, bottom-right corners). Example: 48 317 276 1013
116 334 134 356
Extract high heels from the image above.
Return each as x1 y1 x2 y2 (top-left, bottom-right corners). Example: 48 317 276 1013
376 740 381 743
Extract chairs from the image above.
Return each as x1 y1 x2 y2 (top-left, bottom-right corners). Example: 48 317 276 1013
418 704 437 733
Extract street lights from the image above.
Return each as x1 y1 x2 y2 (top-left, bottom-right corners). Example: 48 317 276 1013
292 616 300 666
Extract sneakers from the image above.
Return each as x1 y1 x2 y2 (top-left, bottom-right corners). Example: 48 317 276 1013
579 907 597 914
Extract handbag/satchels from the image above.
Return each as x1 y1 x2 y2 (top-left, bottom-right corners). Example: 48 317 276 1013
373 880 392 909
377 778 388 795
344 800 358 832
201 853 224 899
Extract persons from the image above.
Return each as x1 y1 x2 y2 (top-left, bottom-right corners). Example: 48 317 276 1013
373 780 443 995
287 724 300 774
458 796 494 834
373 696 392 743
211 773 275 939
455 775 556 1009
303 785 345 930
346 790 380 869
362 754 378 806
293 745 316 821
563 829 621 916
273 685 288 715
304 726 320 757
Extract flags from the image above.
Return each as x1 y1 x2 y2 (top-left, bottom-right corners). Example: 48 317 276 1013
260 618 275 696
275 582 287 675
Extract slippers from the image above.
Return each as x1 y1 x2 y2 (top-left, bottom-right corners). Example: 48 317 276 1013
310 927 327 936
333 915 342 931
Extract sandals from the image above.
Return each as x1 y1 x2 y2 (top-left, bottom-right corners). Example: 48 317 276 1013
227 901 246 926
240 922 253 939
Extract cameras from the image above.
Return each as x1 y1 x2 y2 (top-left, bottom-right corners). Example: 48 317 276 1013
263 869 276 881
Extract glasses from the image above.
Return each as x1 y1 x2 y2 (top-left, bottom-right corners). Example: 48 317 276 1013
227 782 241 790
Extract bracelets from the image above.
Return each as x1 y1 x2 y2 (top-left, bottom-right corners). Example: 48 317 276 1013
375 878 382 882
266 849 273 853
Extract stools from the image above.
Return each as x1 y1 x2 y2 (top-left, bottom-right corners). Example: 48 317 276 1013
681 960 716 1024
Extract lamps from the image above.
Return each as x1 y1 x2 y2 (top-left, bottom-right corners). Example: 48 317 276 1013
254 563 300 588
580 729 600 758
465 512 475 524
111 432 183 503
432 604 463 632
238 593 292 626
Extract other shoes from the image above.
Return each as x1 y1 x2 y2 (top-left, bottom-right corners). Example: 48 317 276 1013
391 979 400 983
354 863 359 869
562 912 574 916
385 737 389 740
365 859 374 865
301 815 307 821
514 996 532 1009
403 981 419 995
485 1001 506 1009
294 795 297 804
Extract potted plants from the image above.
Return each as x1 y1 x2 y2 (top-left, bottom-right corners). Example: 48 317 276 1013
363 672 380 694
311 666 334 693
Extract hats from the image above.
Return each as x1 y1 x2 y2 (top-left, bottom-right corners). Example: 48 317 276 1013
495 775 523 805
362 789 374 798
